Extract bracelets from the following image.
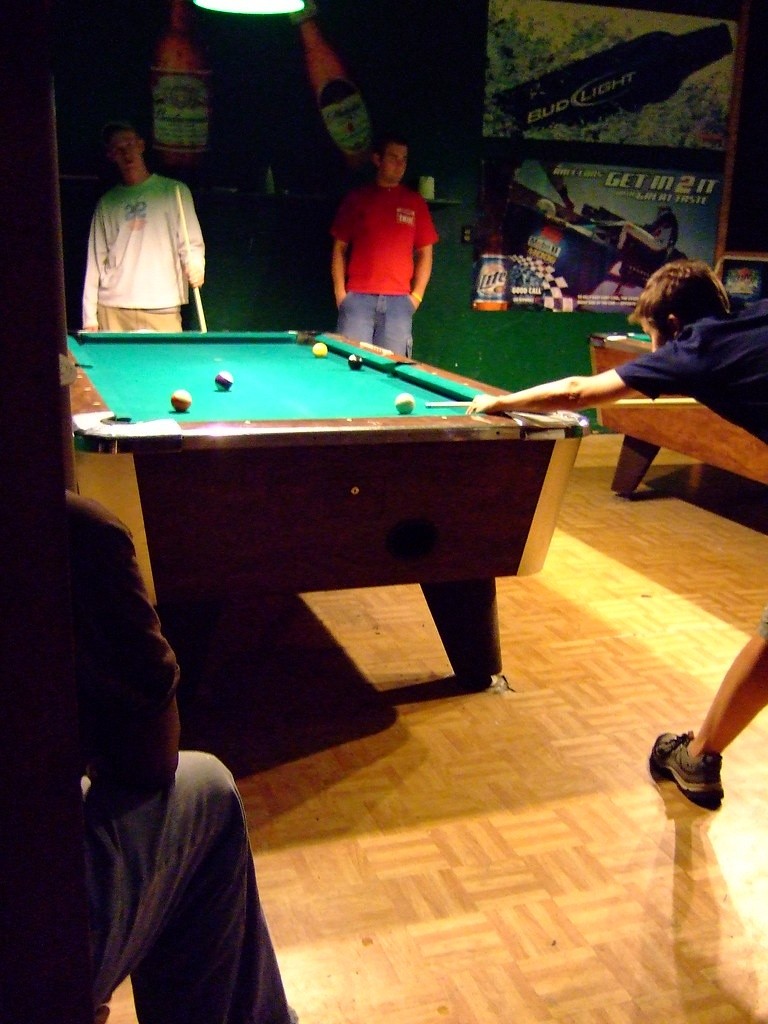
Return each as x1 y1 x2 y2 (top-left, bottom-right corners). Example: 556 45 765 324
411 292 422 302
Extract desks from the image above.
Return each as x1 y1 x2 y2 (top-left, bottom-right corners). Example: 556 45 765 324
69 328 589 686
588 330 768 496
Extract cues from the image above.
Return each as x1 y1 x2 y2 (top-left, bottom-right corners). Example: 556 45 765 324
172 182 209 332
422 394 705 410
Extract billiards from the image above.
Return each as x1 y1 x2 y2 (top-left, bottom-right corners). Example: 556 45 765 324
215 371 234 391
170 390 192 411
347 353 363 370
395 393 415 414
312 342 329 358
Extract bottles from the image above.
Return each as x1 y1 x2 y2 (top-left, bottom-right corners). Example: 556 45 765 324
150 0 213 165
475 235 506 299
492 23 733 129
290 2 373 164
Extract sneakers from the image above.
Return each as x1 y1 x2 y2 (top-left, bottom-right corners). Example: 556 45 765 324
653 733 723 800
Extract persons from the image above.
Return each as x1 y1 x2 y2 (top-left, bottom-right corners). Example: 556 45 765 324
328 132 439 358
71 491 291 1023
81 117 205 334
466 256 768 804
